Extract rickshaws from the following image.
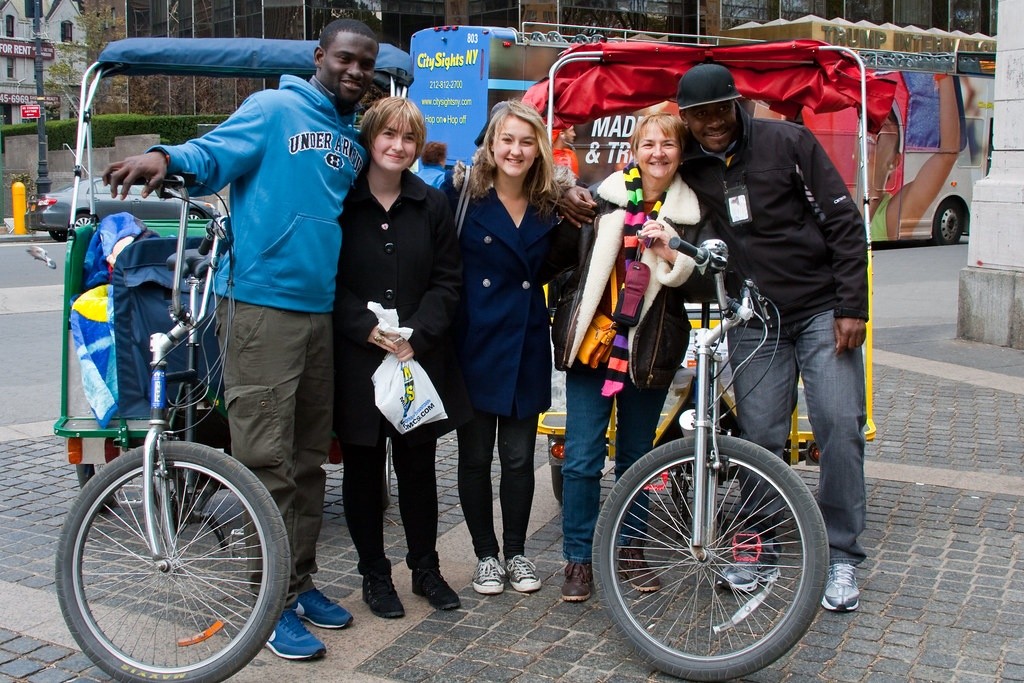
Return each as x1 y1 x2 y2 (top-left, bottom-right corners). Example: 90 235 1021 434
517 37 877 682
53 42 410 683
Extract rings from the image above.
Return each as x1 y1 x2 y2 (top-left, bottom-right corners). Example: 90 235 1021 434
373 336 386 344
377 328 384 335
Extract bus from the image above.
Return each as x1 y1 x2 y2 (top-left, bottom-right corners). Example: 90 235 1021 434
409 14 996 242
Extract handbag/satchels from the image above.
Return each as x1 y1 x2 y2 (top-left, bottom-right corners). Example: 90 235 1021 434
367 301 448 434
576 263 618 369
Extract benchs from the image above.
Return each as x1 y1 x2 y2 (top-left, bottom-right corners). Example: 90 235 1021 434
65 218 210 327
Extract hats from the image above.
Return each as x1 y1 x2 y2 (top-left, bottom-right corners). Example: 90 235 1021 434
677 62 742 109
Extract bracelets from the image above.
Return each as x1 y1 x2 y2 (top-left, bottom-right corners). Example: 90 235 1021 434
147 147 169 165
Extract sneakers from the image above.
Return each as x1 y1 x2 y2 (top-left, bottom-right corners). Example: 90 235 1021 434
561 562 591 600
504 554 541 592
617 546 661 591
362 572 405 616
714 551 782 592
263 608 327 659
473 556 506 594
821 563 860 611
411 566 461 609
291 587 353 628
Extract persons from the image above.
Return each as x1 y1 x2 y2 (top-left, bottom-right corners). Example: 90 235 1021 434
415 141 448 190
102 18 379 662
439 101 582 594
330 96 461 619
868 72 959 240
552 124 578 180
550 112 717 600
558 63 869 611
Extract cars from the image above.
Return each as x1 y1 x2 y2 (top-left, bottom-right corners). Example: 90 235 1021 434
24 177 221 242
